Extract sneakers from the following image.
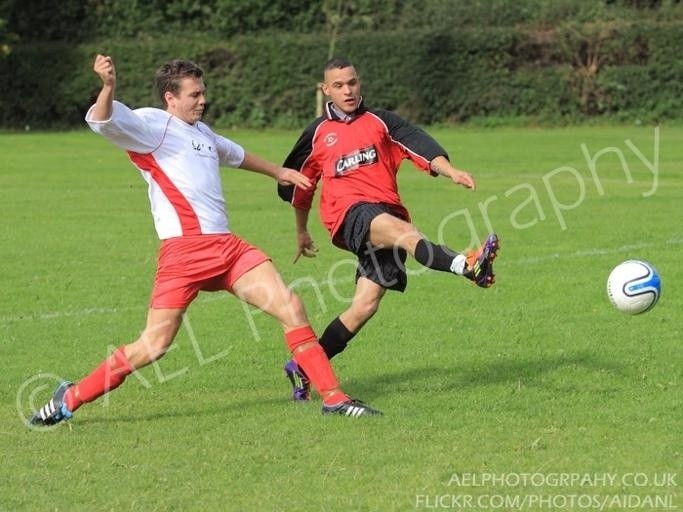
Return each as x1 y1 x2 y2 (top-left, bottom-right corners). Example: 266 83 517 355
28 380 77 427
319 395 384 419
282 358 312 402
462 232 500 290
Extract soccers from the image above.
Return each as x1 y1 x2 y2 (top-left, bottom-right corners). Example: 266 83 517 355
607 260 660 314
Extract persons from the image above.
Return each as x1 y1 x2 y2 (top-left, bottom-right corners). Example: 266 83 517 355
28 55 385 427
276 57 500 403
